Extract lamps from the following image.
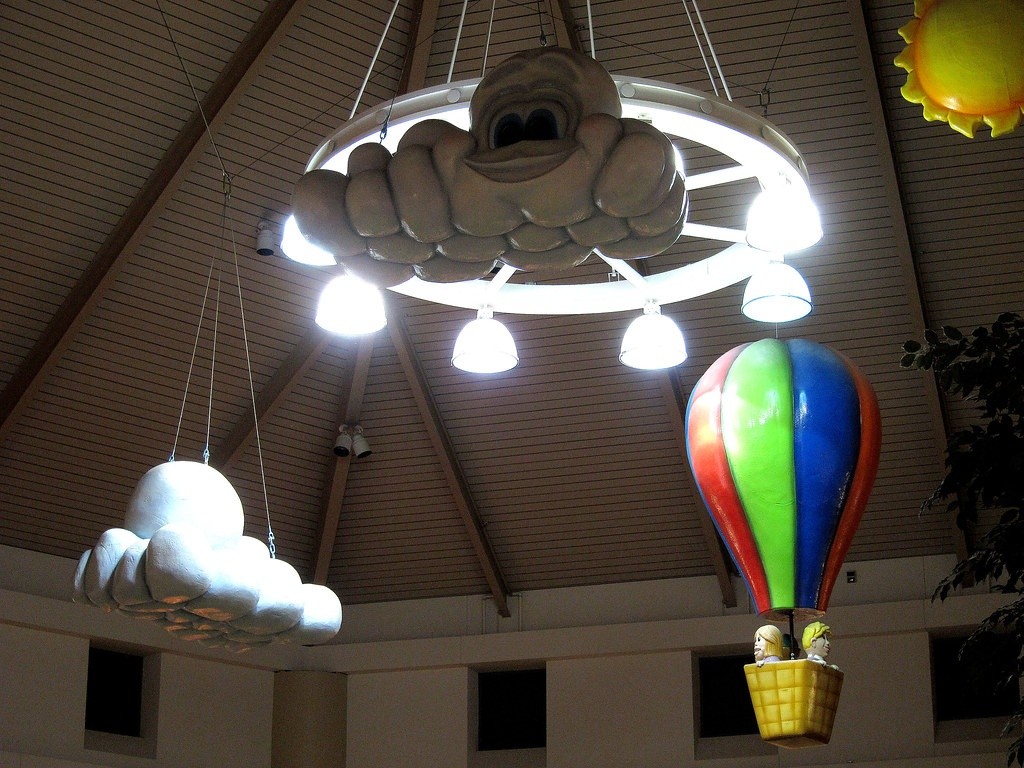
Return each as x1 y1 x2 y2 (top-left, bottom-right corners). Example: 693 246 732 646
279 0 826 380
354 425 372 458
332 424 352 456
256 221 275 257
